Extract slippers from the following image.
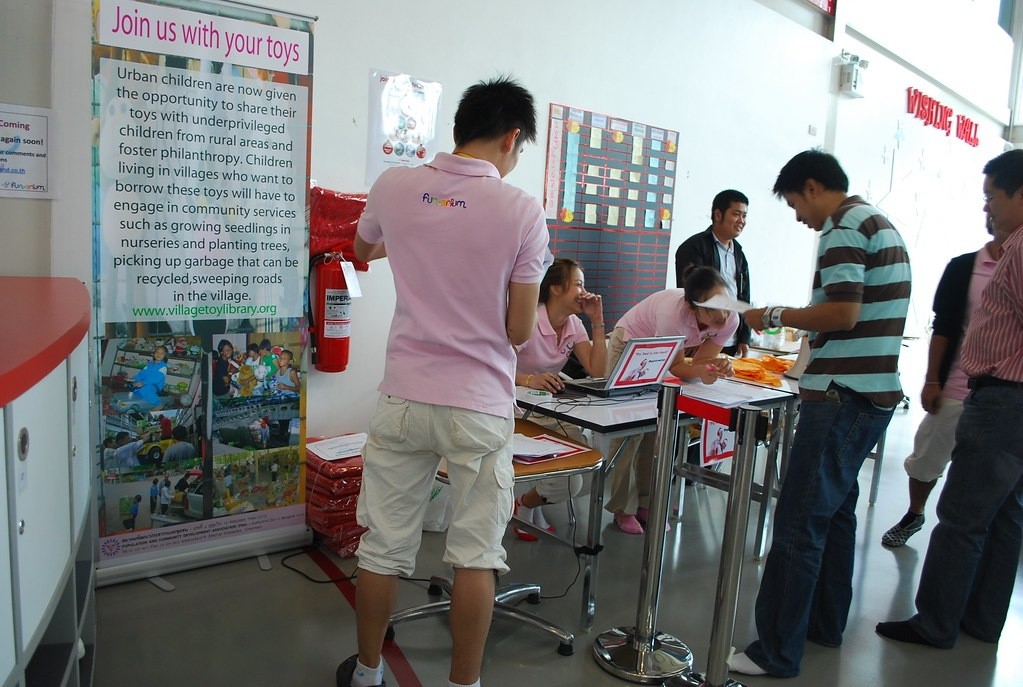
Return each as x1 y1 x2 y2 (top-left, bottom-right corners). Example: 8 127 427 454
882 522 922 547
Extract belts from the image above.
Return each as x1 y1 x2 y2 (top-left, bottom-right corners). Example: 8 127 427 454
967 375 1023 388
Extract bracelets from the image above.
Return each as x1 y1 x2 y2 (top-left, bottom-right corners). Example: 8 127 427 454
925 381 941 385
761 306 773 328
526 374 533 384
591 324 604 329
772 307 785 327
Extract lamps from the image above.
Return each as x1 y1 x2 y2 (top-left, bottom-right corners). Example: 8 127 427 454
840 53 870 100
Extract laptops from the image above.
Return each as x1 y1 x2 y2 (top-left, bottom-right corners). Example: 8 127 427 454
561 335 686 397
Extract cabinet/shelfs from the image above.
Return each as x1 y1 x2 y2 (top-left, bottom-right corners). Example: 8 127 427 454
108 345 202 407
0 277 100 687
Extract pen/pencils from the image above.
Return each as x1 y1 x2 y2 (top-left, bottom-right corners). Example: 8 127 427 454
706 365 715 373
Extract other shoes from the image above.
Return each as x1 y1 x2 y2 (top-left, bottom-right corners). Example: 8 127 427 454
336 653 387 687
512 498 537 541
547 525 554 532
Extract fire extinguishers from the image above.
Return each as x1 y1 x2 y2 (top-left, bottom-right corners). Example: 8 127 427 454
306 249 369 373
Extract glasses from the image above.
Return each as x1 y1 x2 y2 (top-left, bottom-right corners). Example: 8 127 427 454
983 189 1005 203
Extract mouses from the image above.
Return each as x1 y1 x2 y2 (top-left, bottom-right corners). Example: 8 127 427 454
548 384 566 394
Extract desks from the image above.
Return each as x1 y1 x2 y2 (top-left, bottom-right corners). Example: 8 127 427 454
687 334 895 560
385 418 604 657
502 372 795 633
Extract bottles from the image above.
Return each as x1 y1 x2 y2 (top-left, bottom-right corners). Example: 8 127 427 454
760 326 785 350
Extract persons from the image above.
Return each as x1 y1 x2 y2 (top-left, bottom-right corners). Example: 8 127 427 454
212 336 300 401
606 265 742 534
162 425 195 464
725 149 911 678
103 430 151 469
128 345 168 410
333 74 551 687
123 494 142 530
216 459 281 497
515 256 608 544
676 190 750 490
149 473 190 517
159 414 172 440
260 416 271 441
877 145 1023 649
